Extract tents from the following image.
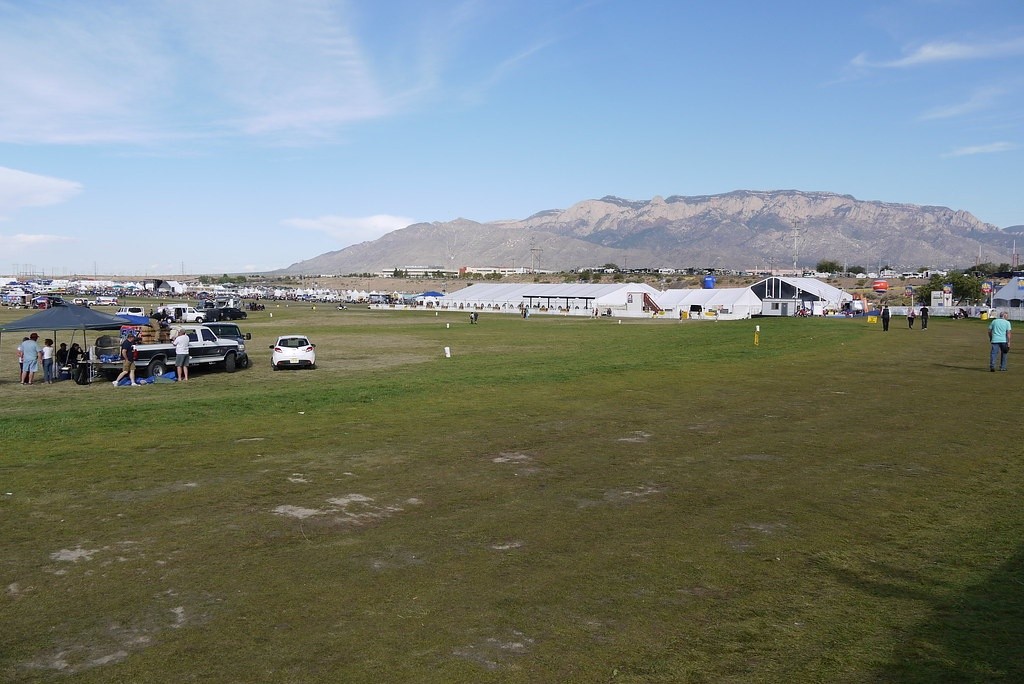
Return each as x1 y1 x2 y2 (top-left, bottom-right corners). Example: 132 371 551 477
0 303 131 380
654 288 763 320
96 314 169 331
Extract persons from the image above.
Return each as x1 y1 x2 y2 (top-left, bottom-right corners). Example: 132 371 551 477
987 311 1011 373
172 328 190 381
523 305 529 318
340 303 344 310
17 337 30 382
112 299 115 302
469 312 479 324
149 309 153 317
249 302 265 311
607 308 611 315
881 305 891 332
56 343 84 366
19 333 42 384
24 288 52 309
279 296 282 300
907 307 915 330
112 334 141 388
920 304 931 331
41 338 57 384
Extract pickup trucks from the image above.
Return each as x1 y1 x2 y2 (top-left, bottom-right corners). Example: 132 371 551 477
89 324 239 378
71 298 96 306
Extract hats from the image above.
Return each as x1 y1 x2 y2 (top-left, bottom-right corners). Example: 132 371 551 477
30 333 40 338
60 343 68 346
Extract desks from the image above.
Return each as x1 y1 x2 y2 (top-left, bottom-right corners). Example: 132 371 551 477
77 360 94 383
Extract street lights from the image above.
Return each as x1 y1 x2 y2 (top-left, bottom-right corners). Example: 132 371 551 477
623 255 628 272
511 258 515 274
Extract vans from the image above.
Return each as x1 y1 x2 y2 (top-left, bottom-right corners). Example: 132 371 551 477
35 287 53 295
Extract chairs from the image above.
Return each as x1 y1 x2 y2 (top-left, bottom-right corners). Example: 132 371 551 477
283 341 288 346
298 341 304 346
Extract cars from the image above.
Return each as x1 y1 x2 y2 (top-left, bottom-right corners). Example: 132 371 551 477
158 295 249 323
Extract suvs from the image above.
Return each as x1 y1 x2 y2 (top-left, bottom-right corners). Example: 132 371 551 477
201 322 252 370
114 306 145 317
268 335 316 371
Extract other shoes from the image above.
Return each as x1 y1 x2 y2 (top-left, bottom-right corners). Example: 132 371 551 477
130 383 141 387
111 381 119 389
41 380 53 385
20 383 25 386
27 382 33 386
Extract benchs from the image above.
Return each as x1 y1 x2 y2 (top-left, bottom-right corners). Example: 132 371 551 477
56 356 73 379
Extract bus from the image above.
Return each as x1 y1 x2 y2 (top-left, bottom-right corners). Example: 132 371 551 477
48 292 62 299
95 297 118 307
